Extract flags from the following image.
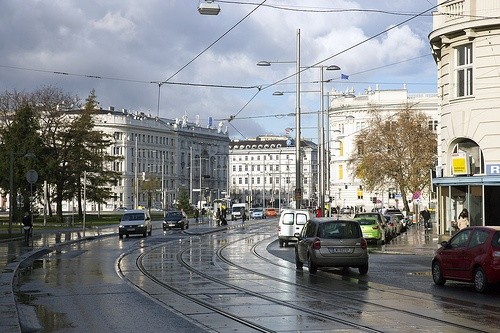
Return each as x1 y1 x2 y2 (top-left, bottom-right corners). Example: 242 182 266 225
341 74 348 79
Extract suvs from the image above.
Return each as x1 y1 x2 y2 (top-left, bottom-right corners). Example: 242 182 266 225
384 209 407 228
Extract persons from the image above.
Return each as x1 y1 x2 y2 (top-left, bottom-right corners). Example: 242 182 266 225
457 210 469 230
216 207 227 226
138 204 142 209
240 207 245 222
22 212 33 247
422 207 431 228
194 208 206 223
317 207 328 217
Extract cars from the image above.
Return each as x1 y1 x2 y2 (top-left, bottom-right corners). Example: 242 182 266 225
251 208 267 219
118 209 150 237
296 217 370 274
163 210 189 230
430 226 499 293
356 217 384 244
266 208 276 217
357 214 403 240
232 202 250 221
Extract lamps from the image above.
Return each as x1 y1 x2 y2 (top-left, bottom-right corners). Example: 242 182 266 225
197 0 221 16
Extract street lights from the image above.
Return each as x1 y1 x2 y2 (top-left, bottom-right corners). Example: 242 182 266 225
259 27 341 209
285 93 354 217
272 66 355 222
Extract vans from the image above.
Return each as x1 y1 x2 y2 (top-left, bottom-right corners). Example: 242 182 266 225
277 209 313 249
